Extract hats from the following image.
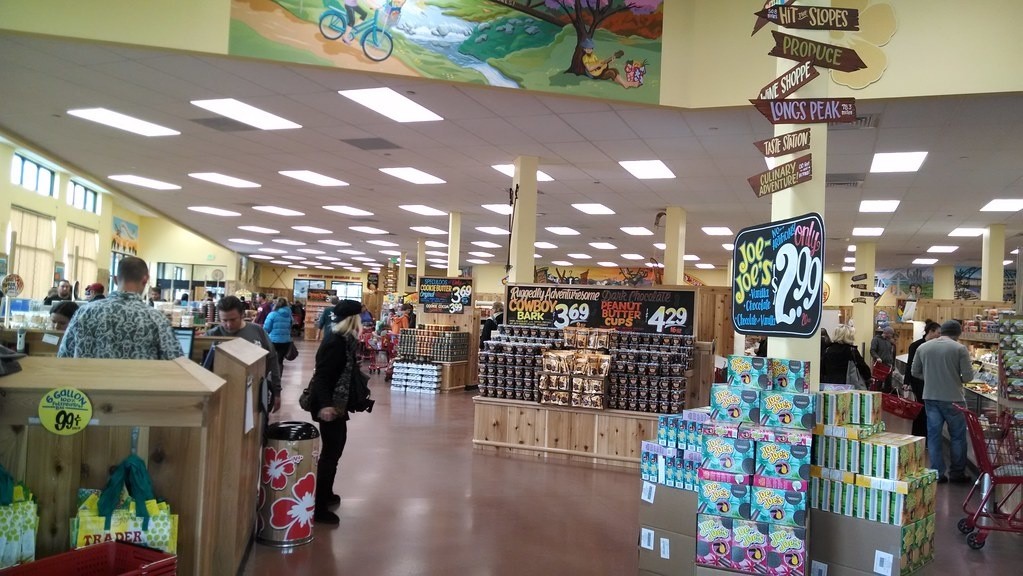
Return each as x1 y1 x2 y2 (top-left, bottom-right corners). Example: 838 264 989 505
883 326 894 334
940 320 961 336
330 299 361 322
87 283 104 292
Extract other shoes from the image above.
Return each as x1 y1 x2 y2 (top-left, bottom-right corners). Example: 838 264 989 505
314 512 340 523
328 495 341 507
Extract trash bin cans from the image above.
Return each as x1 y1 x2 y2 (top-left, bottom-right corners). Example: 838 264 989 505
256 421 320 548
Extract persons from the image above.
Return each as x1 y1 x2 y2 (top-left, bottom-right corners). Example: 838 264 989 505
44 280 416 419
870 326 896 394
202 295 283 416
308 299 376 525
478 301 504 351
911 320 974 485
903 318 941 450
56 257 183 360
821 323 872 391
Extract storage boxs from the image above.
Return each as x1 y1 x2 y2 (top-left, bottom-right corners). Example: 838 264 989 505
422 376 443 382
393 361 408 367
420 387 442 394
391 373 407 379
963 308 1023 429
391 379 407 386
637 354 939 576
390 385 406 392
407 379 422 386
421 382 442 389
393 367 408 373
153 301 195 328
2 299 89 330
423 364 442 370
406 386 421 393
408 362 423 369
408 368 423 375
422 370 442 375
406 373 422 380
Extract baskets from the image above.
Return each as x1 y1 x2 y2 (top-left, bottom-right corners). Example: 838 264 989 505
0 539 177 576
871 361 893 381
882 387 923 420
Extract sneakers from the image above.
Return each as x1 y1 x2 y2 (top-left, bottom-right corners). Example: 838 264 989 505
937 476 948 483
950 473 971 484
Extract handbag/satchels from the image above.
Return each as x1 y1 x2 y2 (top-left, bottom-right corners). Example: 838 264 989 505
846 347 867 390
285 342 298 360
299 362 375 413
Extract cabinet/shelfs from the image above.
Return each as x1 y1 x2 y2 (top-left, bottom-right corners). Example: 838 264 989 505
999 332 1023 463
914 299 1013 326
471 340 717 476
303 302 336 341
700 285 734 357
361 291 385 321
874 285 914 356
416 303 481 386
941 331 1001 467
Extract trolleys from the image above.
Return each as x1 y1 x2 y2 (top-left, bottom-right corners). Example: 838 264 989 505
366 332 391 375
952 402 1023 549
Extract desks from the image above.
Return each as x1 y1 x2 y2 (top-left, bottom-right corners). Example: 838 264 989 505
415 360 469 394
